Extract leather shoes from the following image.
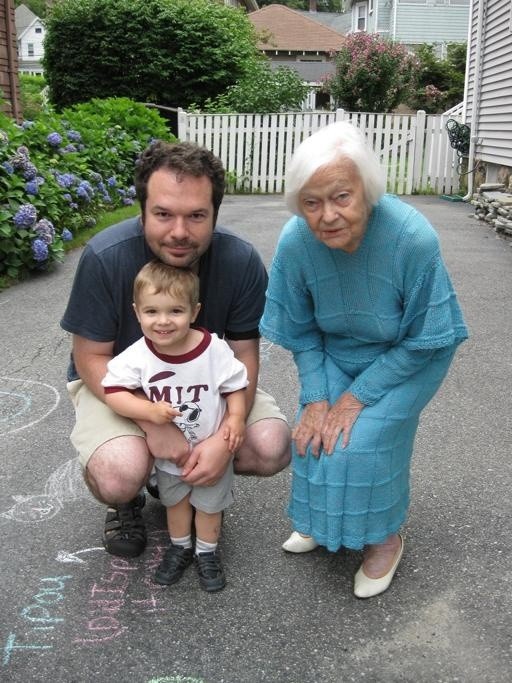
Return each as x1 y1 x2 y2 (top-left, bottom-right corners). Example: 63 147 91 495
281 530 320 553
353 534 404 600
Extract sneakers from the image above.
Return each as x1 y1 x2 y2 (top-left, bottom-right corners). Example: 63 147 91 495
154 542 194 585
101 495 148 559
192 550 226 592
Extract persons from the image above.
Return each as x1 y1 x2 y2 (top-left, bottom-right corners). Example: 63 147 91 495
59 140 295 557
98 258 251 593
256 115 469 602
